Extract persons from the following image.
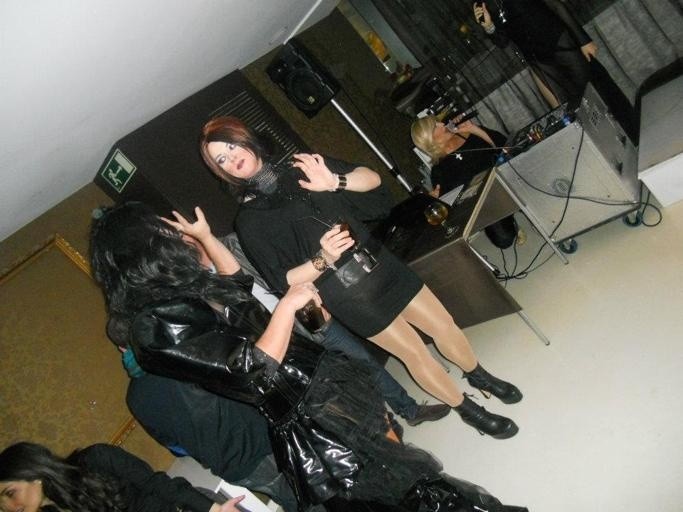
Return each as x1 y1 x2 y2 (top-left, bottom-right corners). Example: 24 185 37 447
198 115 523 439
0 443 245 512
174 209 451 448
409 114 528 250
87 200 530 512
104 312 300 512
472 0 640 149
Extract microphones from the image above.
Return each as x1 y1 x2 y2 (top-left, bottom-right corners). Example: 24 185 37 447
477 0 485 24
446 110 479 130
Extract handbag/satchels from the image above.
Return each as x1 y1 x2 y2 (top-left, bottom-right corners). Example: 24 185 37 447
298 353 386 455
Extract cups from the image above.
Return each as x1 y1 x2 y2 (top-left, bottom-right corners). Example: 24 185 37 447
320 215 361 254
294 299 331 336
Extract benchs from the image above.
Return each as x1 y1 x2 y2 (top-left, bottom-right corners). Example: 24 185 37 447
638 74 683 207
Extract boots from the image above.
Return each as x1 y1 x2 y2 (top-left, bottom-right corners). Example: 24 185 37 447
453 393 517 439
464 363 522 403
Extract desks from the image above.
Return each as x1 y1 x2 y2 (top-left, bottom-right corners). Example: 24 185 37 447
410 1 683 175
166 455 280 512
389 166 568 372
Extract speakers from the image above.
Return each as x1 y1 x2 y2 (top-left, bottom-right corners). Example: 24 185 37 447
265 37 341 120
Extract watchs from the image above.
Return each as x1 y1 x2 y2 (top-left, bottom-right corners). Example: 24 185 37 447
310 251 333 272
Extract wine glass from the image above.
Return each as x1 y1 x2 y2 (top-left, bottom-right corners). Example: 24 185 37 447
423 201 460 239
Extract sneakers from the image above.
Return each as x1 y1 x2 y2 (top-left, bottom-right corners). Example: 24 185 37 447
406 402 450 427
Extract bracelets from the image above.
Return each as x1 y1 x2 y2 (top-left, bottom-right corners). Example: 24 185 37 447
328 172 347 193
